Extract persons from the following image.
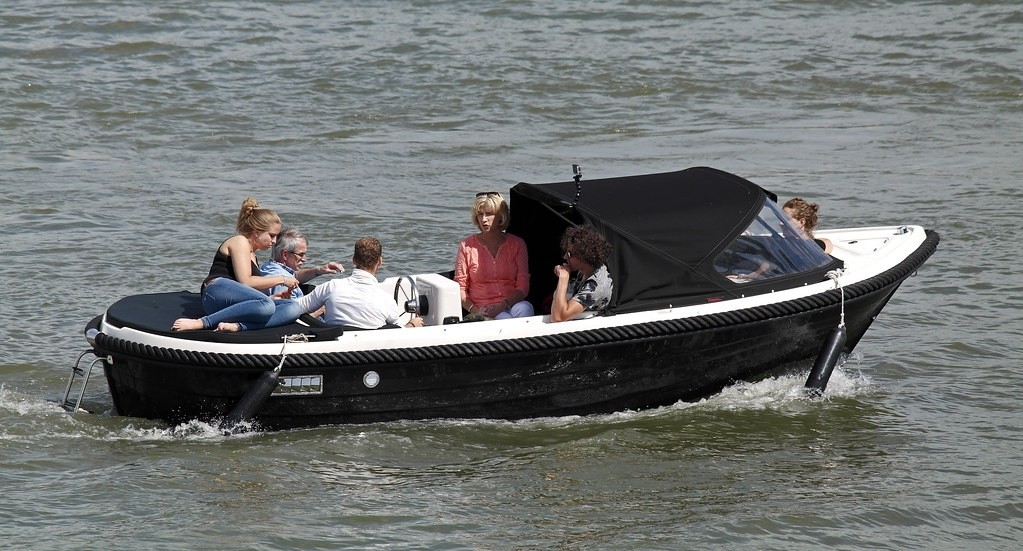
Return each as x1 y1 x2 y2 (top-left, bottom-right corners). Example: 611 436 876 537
550 227 614 323
170 199 303 335
452 194 534 319
262 227 346 323
737 198 825 283
294 236 423 328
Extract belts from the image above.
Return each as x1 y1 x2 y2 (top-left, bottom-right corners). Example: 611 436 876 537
201 277 214 303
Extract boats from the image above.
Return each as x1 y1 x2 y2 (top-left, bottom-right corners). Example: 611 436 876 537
60 165 943 434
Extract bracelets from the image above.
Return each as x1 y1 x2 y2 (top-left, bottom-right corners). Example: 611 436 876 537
315 266 321 276
281 275 286 287
468 304 473 312
406 322 415 327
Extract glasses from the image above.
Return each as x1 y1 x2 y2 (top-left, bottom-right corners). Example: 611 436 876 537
476 191 500 197
565 249 578 258
282 248 308 259
